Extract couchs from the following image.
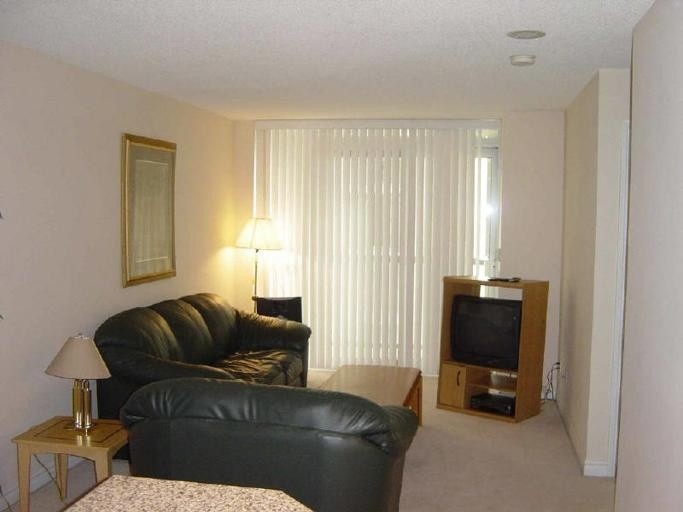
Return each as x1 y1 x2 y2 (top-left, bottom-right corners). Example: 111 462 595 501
119 377 419 511
93 293 311 461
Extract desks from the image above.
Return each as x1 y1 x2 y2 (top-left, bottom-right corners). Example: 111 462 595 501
10 415 129 512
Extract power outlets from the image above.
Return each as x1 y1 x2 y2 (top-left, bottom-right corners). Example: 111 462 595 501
555 353 567 378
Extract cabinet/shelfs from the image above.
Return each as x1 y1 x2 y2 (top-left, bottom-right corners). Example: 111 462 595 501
436 274 549 424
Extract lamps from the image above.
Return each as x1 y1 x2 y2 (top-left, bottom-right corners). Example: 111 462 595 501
44 334 113 430
236 218 283 313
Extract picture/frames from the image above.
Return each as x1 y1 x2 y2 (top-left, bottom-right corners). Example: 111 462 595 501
119 132 177 289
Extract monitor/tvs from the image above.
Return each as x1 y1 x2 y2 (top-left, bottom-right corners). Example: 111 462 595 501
450 295 522 372
